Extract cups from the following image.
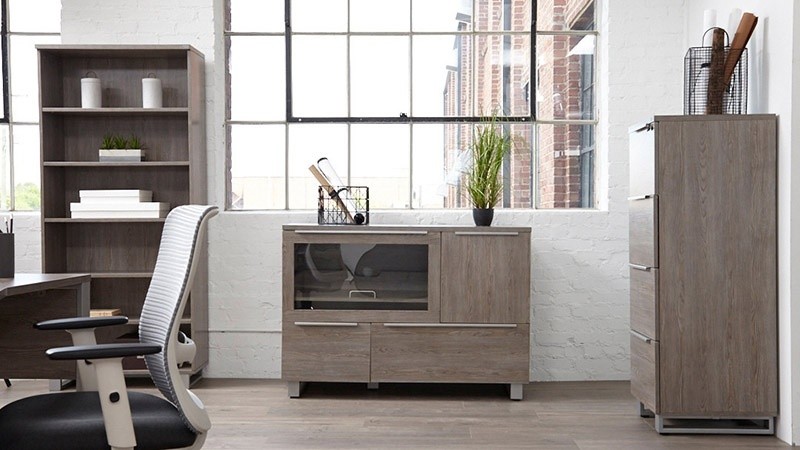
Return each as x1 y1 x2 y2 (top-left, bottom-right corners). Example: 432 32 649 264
0 233 15 279
141 77 163 109
80 78 102 109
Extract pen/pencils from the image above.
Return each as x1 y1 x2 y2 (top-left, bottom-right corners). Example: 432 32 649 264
10 212 13 233
4 216 9 233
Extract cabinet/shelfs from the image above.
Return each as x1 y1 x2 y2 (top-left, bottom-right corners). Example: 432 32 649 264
627 114 779 434
34 42 208 393
282 223 533 401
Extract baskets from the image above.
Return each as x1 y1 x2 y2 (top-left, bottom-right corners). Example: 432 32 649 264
683 28 748 116
317 185 370 225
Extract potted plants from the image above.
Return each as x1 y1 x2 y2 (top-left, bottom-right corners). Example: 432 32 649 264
459 102 536 227
99 130 146 161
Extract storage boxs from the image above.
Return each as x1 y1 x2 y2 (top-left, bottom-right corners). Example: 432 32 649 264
70 188 170 219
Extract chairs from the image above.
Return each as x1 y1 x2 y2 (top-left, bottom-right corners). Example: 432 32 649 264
0 204 220 450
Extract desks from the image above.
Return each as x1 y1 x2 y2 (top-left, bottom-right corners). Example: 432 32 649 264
0 272 91 388
